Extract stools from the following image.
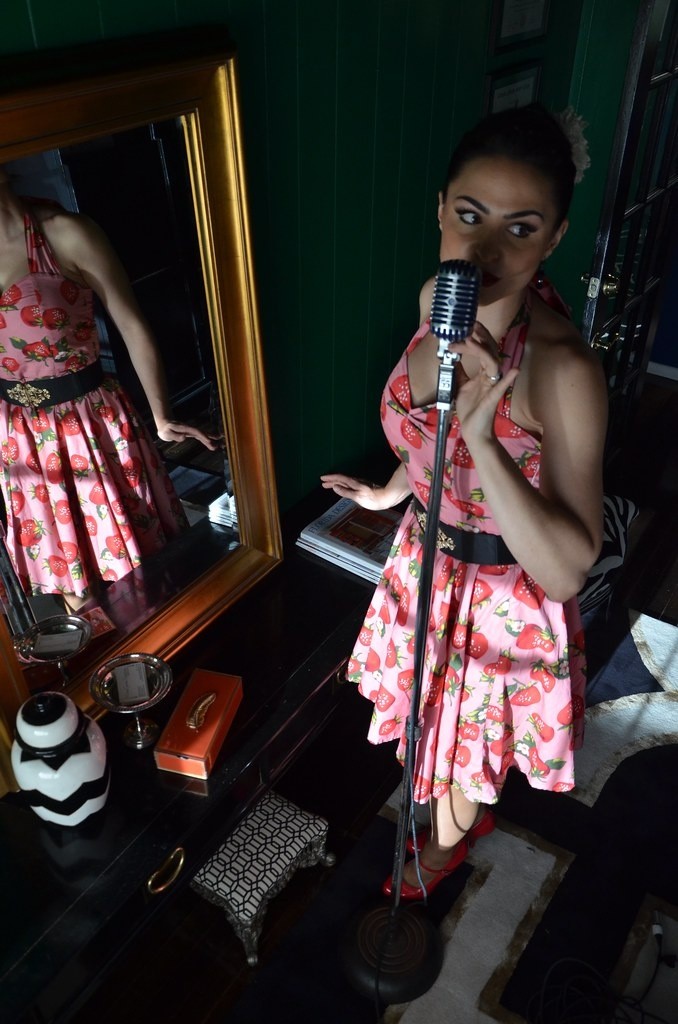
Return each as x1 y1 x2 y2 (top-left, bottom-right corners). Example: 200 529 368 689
192 788 334 967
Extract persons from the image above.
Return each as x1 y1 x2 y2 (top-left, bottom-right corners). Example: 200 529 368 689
0 198 215 647
317 106 609 899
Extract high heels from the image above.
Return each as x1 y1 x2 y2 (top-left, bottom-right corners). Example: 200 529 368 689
405 809 496 855
383 839 468 900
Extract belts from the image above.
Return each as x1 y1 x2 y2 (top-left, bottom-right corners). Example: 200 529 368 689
0 359 105 407
412 495 516 567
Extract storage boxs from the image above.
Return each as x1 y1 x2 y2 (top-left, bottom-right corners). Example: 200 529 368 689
153 669 243 780
14 600 116 664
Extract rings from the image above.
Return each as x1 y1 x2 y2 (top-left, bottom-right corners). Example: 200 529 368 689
484 372 501 380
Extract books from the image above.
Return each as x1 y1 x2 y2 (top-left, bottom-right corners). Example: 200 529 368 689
209 493 237 527
295 496 404 584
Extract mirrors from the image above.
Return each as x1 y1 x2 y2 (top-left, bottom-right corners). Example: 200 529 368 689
0 56 284 800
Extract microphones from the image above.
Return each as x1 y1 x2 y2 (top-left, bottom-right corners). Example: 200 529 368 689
429 259 483 409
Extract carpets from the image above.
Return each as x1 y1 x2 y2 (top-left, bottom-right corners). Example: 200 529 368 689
220 596 677 1023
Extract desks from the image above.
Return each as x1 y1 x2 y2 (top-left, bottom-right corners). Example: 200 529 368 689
0 546 383 1024
